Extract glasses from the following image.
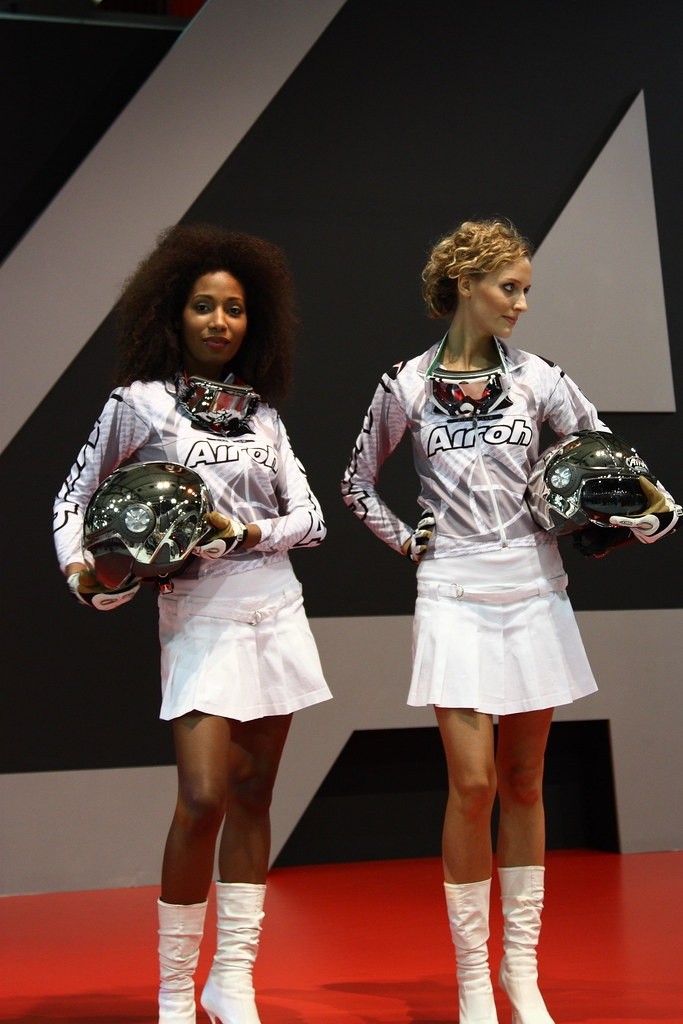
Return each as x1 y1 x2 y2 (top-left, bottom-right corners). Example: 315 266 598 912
177 376 260 435
426 368 507 415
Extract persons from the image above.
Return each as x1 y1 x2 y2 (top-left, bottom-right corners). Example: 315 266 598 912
341 218 683 1024
50 225 333 1023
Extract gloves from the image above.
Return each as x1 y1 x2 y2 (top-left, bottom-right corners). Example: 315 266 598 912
191 511 247 561
66 569 142 612
610 476 683 545
407 509 435 565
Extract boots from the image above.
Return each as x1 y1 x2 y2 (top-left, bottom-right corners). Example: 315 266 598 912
157 896 208 1024
200 880 266 1024
498 866 555 1024
444 877 498 1024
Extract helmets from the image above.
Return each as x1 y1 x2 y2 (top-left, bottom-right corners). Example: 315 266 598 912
524 429 656 540
82 461 215 590
